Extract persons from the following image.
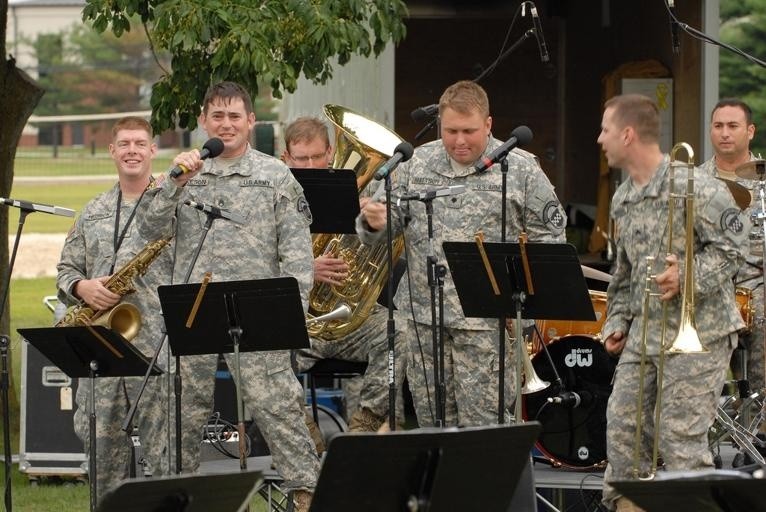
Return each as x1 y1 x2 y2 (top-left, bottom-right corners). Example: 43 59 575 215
282 117 406 458
137 80 322 510
354 79 567 430
694 101 766 473
56 116 177 508
598 94 751 512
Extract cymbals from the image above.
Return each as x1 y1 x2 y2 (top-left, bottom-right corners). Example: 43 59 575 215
714 176 751 211
735 160 766 180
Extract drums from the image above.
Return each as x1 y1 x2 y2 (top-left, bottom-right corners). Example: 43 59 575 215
732 286 754 330
522 289 620 471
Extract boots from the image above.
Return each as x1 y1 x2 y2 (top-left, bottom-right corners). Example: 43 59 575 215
349 407 383 434
615 496 646 512
292 489 314 512
306 422 328 458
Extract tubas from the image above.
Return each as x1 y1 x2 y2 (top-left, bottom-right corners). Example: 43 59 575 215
289 103 404 340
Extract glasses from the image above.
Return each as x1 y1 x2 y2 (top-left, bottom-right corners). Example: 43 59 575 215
287 145 330 162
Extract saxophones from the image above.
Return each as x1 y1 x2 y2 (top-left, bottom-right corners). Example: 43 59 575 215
52 235 172 343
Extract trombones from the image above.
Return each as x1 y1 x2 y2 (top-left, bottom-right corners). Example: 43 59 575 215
633 142 709 481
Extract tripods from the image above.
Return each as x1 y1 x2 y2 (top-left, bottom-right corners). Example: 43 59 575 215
708 332 766 472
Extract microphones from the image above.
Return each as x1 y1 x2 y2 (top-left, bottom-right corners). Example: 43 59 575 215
412 103 440 122
668 0 681 57
170 137 224 178
374 141 413 181
186 200 246 224
530 7 550 64
401 185 464 201
476 126 534 173
5 198 76 218
557 391 592 407
